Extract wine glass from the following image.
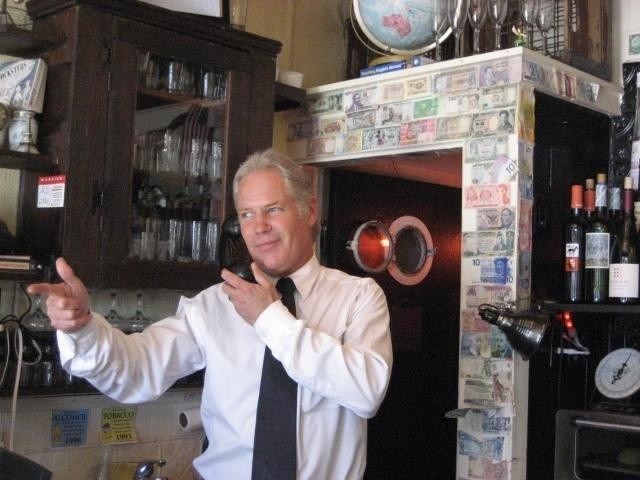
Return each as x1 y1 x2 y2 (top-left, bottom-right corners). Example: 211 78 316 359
428 0 553 63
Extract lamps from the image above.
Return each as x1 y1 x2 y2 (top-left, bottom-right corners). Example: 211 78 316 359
478 302 592 362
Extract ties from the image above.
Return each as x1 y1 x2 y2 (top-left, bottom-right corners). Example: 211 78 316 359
251 277 298 479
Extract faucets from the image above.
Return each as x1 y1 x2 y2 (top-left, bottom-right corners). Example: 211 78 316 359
132 457 166 480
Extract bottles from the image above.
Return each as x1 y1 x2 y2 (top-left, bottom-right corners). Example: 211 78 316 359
18 285 159 393
564 173 639 305
10 110 41 156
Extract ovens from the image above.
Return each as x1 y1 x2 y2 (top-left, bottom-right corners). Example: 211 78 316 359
555 409 639 479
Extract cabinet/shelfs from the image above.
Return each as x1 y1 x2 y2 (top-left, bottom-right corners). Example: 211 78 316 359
1 25 65 280
20 0 285 288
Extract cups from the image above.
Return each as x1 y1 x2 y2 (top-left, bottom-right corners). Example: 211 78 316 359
127 50 228 263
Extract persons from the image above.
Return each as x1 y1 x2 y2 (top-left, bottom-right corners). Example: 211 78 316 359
29 149 396 480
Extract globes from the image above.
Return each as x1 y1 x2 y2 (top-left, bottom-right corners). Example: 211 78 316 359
350 0 462 67
